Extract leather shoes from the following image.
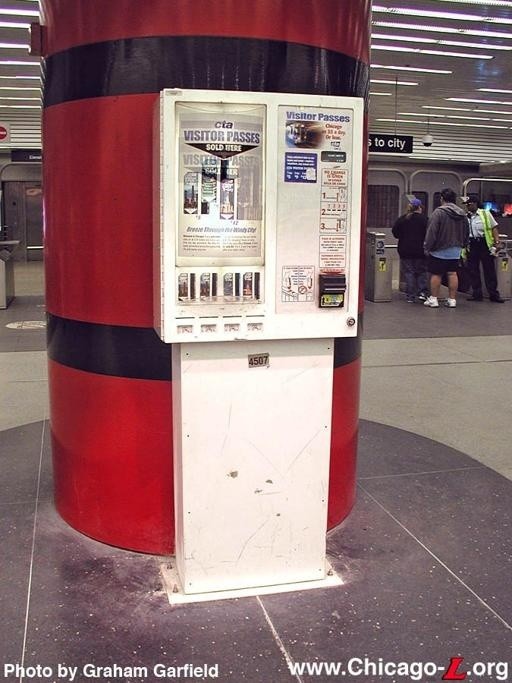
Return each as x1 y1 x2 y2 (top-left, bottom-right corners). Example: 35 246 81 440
465 294 483 301
488 294 505 303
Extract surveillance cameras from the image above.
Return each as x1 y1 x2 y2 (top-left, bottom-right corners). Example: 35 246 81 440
421 136 433 146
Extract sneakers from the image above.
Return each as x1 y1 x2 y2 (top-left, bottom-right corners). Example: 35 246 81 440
423 295 439 308
417 291 428 300
405 295 416 302
443 296 457 308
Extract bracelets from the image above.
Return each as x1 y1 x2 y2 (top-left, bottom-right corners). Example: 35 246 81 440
495 241 500 245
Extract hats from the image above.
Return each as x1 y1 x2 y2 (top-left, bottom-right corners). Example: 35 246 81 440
462 196 478 204
409 197 422 208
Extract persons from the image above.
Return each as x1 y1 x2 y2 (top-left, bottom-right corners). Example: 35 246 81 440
463 195 505 303
392 200 431 303
423 187 470 308
